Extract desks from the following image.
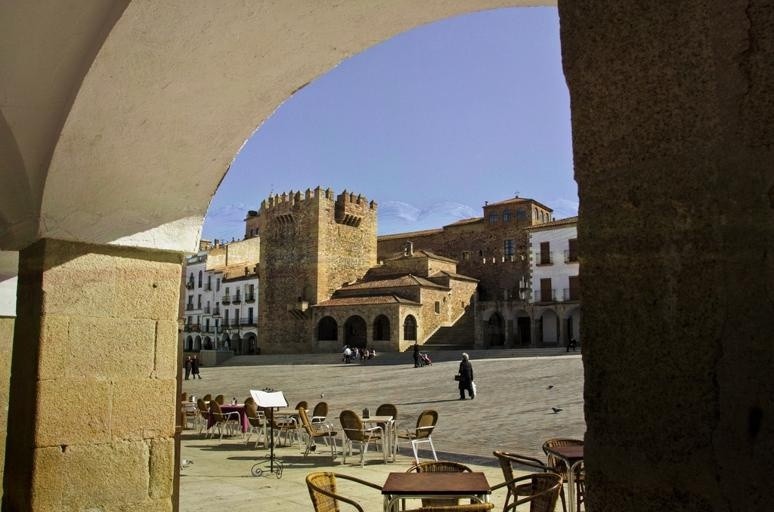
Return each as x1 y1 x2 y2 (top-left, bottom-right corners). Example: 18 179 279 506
382 472 491 509
546 445 584 511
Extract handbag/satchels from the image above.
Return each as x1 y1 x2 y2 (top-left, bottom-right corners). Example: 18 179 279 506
455 373 463 381
471 381 477 397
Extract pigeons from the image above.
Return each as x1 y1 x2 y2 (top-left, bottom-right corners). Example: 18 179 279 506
551 406 564 415
320 392 324 397
547 385 554 390
179 459 193 471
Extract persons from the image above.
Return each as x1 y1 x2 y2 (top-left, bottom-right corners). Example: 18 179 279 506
566 337 572 352
341 345 375 364
573 337 577 351
192 354 202 379
185 356 192 380
458 353 474 400
413 344 420 368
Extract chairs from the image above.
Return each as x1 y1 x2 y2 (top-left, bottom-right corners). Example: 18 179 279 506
179 391 438 468
492 449 567 512
401 502 494 512
402 462 474 510
486 472 564 512
306 471 394 512
543 437 585 483
576 466 586 512
